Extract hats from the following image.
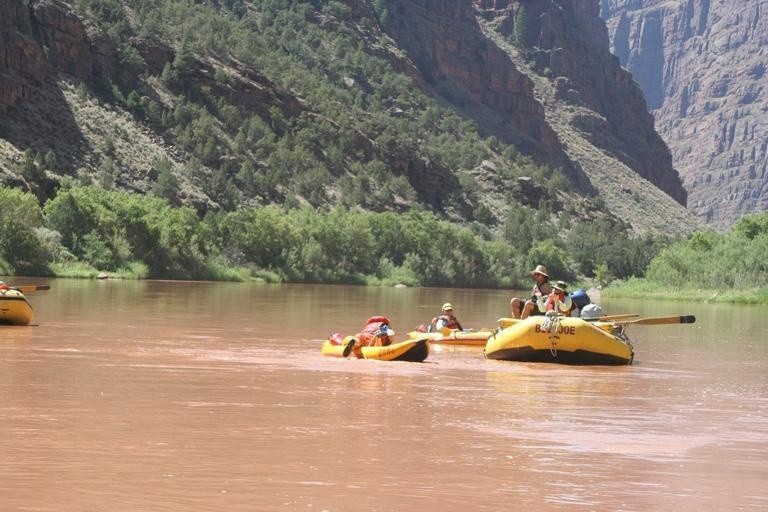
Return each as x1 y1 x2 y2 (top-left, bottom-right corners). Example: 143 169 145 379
530 264 549 278
551 280 567 291
441 303 455 311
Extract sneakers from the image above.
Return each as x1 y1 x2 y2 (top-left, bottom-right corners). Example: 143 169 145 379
379 323 390 335
342 339 355 358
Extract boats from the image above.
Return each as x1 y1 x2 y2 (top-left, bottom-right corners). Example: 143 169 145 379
0 289 35 325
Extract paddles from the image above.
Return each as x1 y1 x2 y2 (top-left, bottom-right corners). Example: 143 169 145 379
8 286 50 291
441 327 472 336
616 315 695 325
582 314 640 321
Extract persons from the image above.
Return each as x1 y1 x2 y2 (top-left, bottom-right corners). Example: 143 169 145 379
534 279 573 318
344 314 395 359
510 265 553 319
431 302 464 337
